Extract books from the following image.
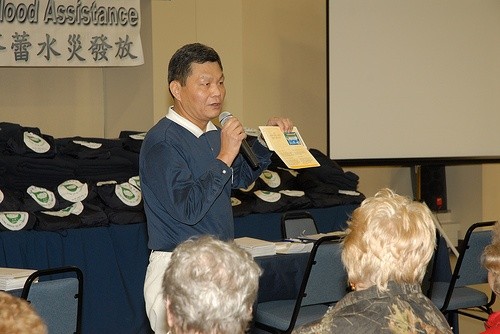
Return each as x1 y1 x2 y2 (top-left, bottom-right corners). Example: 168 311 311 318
0 268 39 292
235 236 276 257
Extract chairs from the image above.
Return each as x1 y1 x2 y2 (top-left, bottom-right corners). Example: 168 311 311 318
255 234 352 334
22 266 83 334
428 221 498 321
279 213 318 244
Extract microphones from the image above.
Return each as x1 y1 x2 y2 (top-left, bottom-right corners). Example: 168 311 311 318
218 111 261 171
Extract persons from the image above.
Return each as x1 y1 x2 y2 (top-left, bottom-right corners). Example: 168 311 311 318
164 234 262 334
293 188 456 334
0 291 48 334
139 42 294 334
481 223 500 334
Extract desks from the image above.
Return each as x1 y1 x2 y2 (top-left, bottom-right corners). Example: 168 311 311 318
253 236 452 303
